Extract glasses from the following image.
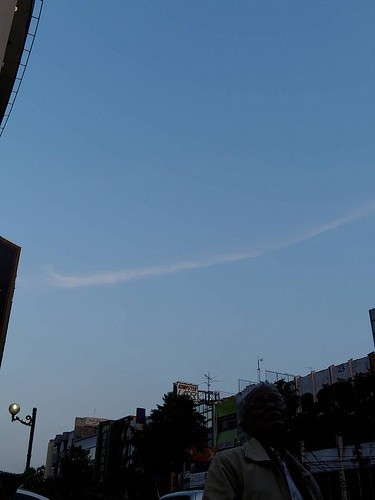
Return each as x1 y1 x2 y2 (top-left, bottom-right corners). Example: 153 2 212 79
245 393 287 409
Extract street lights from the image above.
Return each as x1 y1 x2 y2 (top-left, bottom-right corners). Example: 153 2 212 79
9 402 36 470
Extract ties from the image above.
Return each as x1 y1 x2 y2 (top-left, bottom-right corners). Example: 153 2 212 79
274 450 304 500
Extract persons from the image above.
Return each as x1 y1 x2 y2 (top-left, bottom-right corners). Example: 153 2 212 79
202 381 324 500
